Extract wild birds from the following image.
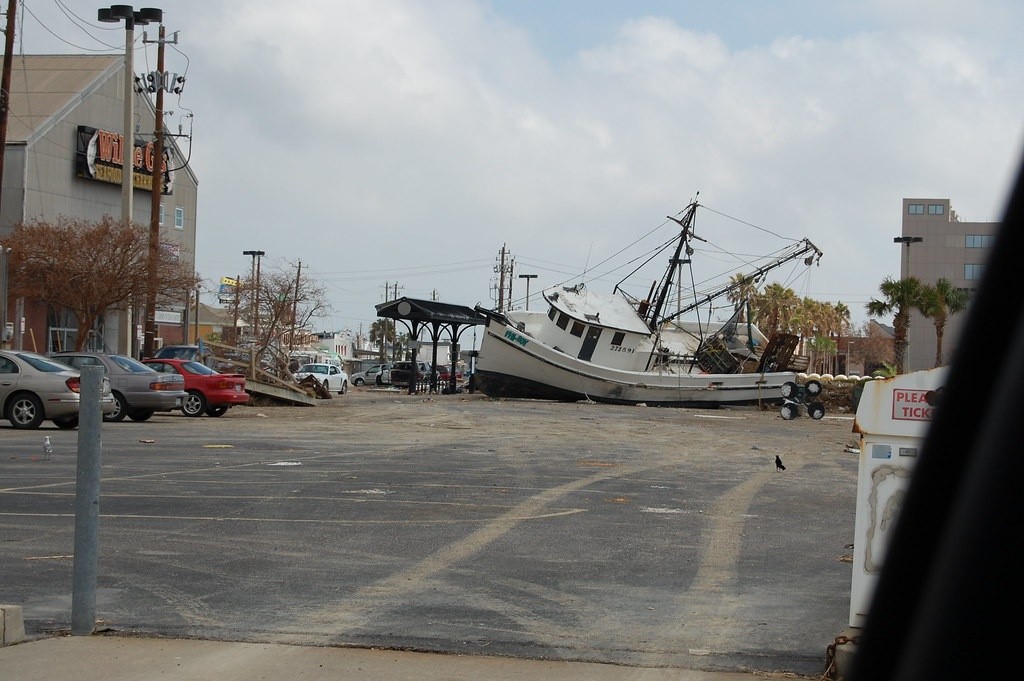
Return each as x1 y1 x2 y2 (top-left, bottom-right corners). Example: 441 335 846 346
43 436 54 460
774 454 786 472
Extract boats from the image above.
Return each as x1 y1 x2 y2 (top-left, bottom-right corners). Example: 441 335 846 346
472 190 827 422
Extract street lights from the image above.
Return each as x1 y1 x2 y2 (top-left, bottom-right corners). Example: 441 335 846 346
518 274 538 311
847 340 855 377
242 250 265 334
893 235 924 374
98 5 164 362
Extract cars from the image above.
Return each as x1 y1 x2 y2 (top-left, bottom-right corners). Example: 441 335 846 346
141 358 250 417
1 348 117 431
350 360 462 388
145 345 215 374
292 363 348 396
45 351 189 424
